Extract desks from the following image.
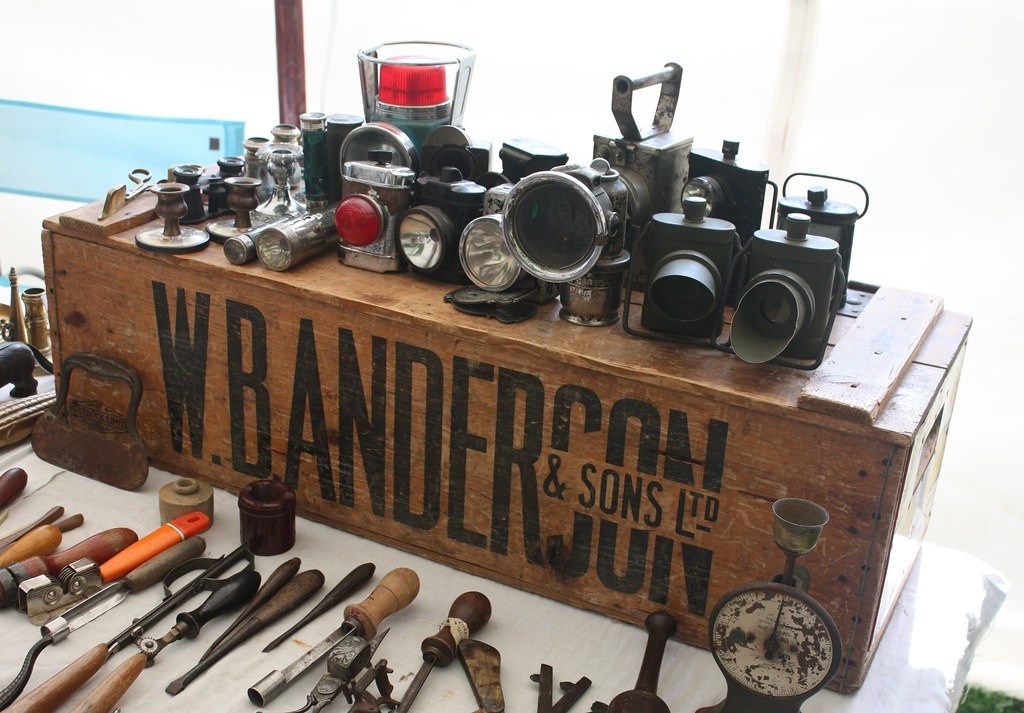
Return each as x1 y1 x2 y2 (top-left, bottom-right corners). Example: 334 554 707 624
0 368 1010 713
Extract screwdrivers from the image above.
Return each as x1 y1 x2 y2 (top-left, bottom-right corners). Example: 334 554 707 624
164 568 325 696
262 562 376 654
198 556 302 664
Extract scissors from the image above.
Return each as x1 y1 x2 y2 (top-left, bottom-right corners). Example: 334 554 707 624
97 168 169 221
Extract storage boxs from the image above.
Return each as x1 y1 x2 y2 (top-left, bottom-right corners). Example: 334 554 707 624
43 184 977 695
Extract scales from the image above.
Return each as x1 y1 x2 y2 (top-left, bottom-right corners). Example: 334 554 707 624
694 498 843 713
